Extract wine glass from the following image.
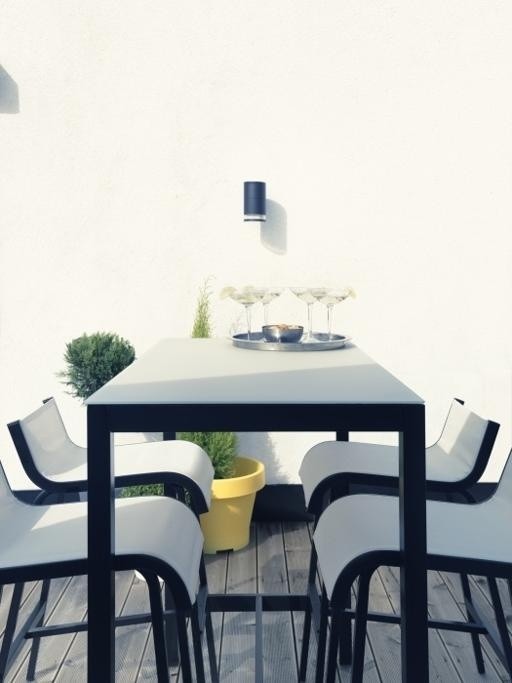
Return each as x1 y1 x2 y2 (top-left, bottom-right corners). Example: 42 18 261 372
291 286 351 342
230 286 284 340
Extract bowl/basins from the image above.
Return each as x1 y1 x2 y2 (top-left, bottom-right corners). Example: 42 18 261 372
262 323 304 343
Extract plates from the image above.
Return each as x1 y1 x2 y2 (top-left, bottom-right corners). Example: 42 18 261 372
230 333 353 351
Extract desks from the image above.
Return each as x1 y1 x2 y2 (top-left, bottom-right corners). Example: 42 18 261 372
84 336 429 683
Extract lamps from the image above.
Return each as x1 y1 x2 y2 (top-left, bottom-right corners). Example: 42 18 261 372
243 181 266 222
